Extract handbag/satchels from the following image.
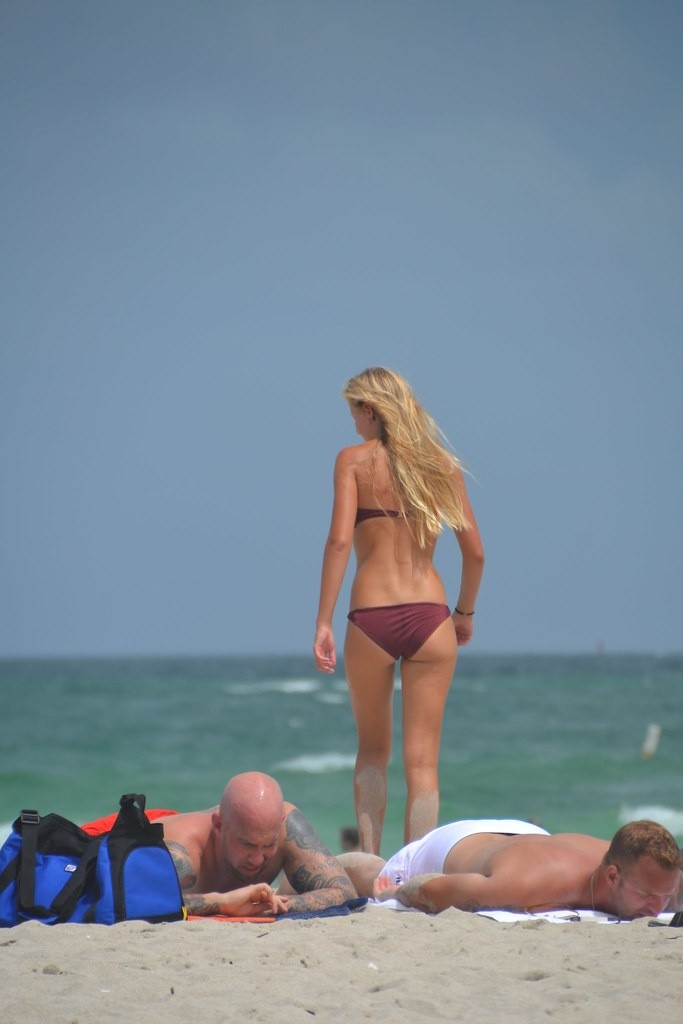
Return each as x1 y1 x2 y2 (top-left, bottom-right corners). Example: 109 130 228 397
0 793 188 928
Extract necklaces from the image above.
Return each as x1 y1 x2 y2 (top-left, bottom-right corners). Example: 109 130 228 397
590 875 596 910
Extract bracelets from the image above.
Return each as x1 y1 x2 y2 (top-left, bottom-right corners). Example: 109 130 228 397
455 607 475 615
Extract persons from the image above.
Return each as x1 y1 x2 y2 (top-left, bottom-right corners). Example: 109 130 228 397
275 816 683 920
340 826 361 852
311 367 484 857
149 771 359 915
